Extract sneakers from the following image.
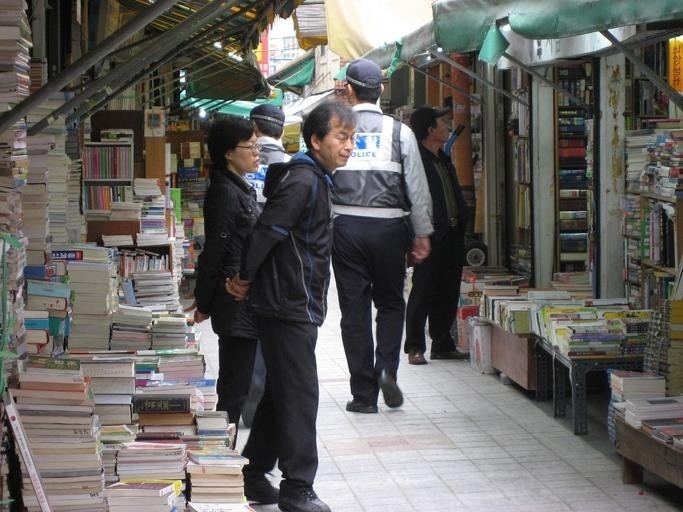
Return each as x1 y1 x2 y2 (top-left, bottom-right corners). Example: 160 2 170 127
278 479 331 512
241 466 281 504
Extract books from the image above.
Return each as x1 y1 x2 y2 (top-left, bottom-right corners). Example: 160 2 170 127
625 41 681 309
669 34 682 121
557 60 592 272
502 68 532 272
2 2 249 511
462 266 656 359
608 300 683 448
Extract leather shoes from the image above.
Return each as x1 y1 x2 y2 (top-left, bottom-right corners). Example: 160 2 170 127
346 399 378 414
408 350 428 365
430 349 470 360
378 367 404 408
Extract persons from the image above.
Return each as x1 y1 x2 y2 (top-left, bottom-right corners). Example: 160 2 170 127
403 106 470 365
323 59 436 413
223 104 357 512
241 104 295 217
194 115 259 450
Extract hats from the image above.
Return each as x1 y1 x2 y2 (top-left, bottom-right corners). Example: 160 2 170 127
410 104 450 140
344 57 384 89
249 104 286 127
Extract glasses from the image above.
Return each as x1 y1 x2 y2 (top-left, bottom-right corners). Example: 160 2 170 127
236 142 263 151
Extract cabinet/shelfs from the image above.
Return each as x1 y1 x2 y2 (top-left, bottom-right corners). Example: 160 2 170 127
593 21 681 496
83 105 174 272
406 21 529 399
529 21 593 436
